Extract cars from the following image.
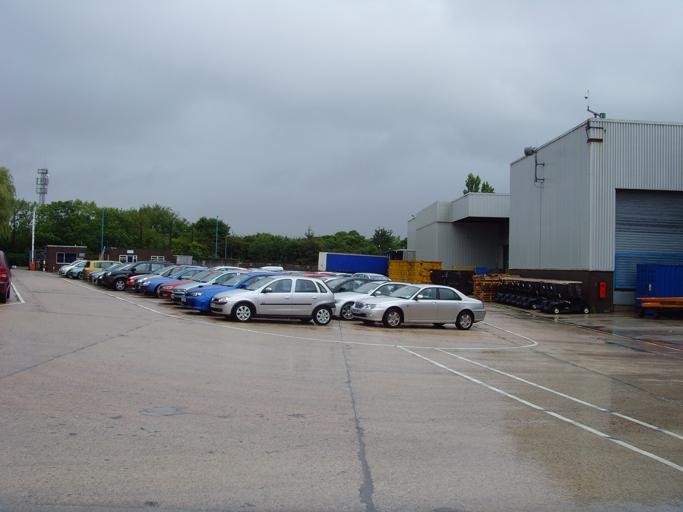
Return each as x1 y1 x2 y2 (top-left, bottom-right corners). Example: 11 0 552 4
59 259 392 315
210 275 336 326
350 284 487 330
0 250 12 303
331 280 413 321
